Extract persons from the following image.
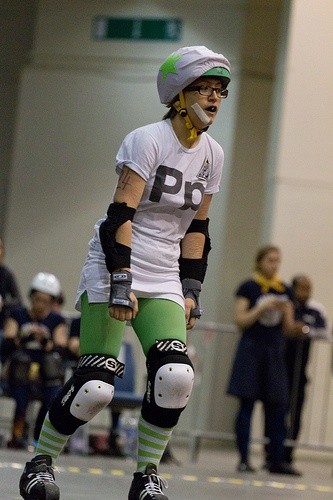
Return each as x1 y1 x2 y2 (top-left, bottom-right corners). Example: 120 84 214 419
225 244 303 472
19 45 232 500
0 239 82 455
262 274 327 476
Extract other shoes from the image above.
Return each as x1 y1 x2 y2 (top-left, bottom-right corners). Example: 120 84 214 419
266 456 270 468
269 463 292 474
282 461 294 473
239 463 253 472
65 441 71 453
161 453 175 464
89 437 96 454
108 432 124 456
12 421 27 448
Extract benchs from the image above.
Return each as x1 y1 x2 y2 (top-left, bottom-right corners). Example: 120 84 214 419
1 342 140 453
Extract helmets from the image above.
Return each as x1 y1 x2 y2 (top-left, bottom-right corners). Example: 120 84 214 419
29 272 60 298
157 46 231 104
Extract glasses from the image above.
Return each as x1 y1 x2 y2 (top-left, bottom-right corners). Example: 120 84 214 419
182 86 228 99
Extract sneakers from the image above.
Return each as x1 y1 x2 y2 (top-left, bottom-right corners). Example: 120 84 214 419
20 455 59 500
128 463 168 500
